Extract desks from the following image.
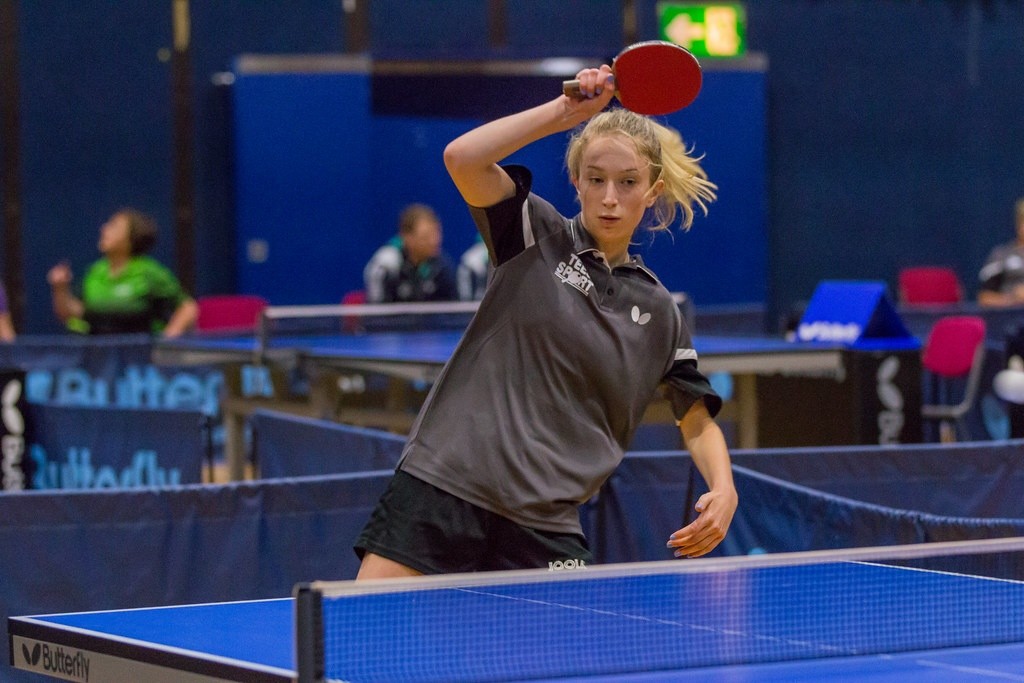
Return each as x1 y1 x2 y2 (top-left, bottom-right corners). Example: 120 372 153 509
5 560 1024 683
890 303 1024 444
147 290 849 484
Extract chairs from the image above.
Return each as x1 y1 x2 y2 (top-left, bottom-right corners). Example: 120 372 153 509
917 316 987 443
898 266 969 302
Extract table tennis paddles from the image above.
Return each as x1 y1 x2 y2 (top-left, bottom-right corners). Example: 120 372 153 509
564 40 705 112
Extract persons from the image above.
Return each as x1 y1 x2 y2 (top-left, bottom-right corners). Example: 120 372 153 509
363 205 493 303
354 64 740 582
0 208 199 340
980 200 1024 308
